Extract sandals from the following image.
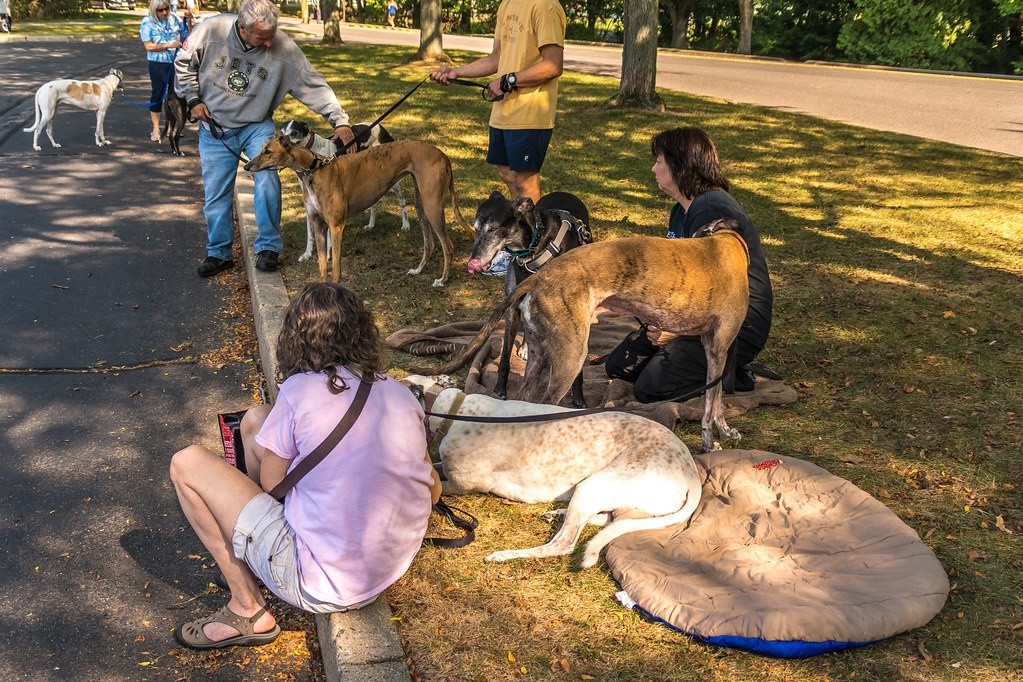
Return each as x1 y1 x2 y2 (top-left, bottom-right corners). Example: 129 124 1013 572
176 603 281 649
217 570 266 589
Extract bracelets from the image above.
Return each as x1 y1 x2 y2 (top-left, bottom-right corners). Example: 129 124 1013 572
500 74 511 94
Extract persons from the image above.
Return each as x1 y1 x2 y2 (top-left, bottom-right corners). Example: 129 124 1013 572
174 0 358 279
0 0 13 35
305 0 324 23
387 0 398 28
140 0 198 142
430 0 567 277
606 126 783 403
170 283 443 649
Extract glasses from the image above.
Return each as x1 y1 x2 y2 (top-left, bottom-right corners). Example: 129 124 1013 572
157 6 169 12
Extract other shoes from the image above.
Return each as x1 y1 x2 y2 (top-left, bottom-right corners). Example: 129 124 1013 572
150 129 161 142
744 363 783 380
166 129 184 137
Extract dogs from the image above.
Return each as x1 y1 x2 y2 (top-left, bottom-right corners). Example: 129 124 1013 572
242 116 754 571
158 92 190 156
23 67 124 151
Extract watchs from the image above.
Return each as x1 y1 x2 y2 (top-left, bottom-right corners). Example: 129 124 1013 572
508 72 518 91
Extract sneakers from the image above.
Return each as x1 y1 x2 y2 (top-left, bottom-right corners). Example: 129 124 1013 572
198 256 234 276
482 251 511 276
256 250 278 270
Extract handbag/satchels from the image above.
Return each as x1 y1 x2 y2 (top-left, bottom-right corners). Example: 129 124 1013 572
217 409 251 473
605 315 660 383
